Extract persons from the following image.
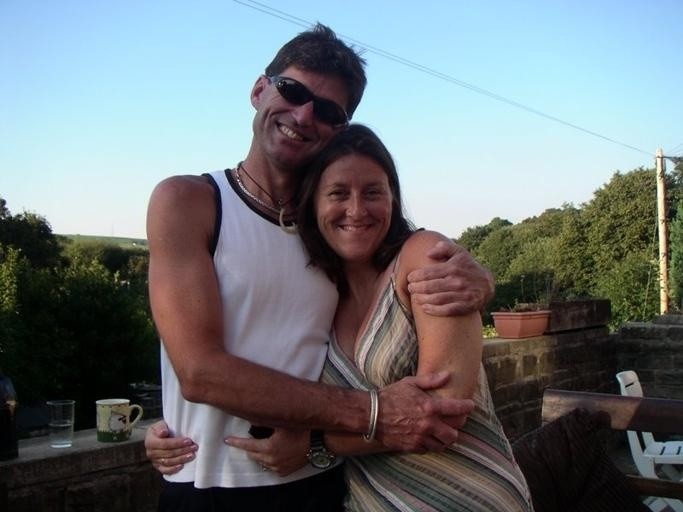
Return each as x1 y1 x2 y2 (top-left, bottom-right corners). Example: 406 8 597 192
144 19 494 511
142 122 537 511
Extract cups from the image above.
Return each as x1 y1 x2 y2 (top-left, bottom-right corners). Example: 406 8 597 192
46 400 75 449
95 398 144 442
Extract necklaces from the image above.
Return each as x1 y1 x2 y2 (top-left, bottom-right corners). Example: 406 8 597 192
241 166 297 234
233 161 301 218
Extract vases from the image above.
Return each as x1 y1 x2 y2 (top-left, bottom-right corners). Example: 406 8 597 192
490 308 553 339
515 298 612 333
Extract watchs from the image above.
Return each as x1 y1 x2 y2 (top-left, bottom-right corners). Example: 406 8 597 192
305 428 336 469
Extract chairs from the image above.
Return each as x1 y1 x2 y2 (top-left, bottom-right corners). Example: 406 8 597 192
512 406 683 512
614 369 683 512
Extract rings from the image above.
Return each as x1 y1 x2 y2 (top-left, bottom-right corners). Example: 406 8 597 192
160 456 165 464
258 461 267 472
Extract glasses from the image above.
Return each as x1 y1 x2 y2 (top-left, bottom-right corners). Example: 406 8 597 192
265 75 348 128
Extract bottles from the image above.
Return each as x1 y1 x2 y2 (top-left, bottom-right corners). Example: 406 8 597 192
0 345 19 461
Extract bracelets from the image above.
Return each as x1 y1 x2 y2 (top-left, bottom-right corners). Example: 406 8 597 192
360 388 379 443
